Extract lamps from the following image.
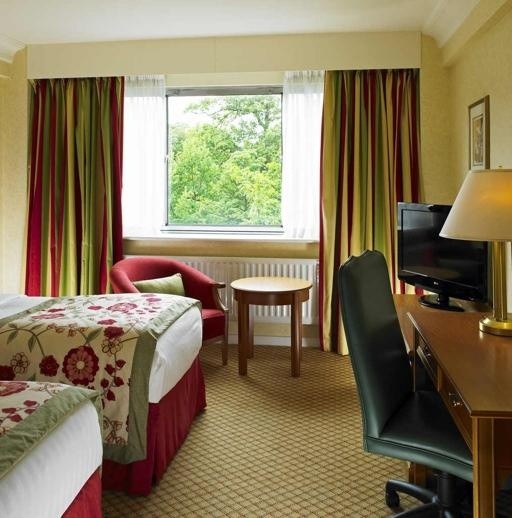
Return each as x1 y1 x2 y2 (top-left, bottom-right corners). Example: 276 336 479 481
438 167 511 338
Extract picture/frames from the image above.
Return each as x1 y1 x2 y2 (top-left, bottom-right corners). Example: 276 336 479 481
467 95 490 170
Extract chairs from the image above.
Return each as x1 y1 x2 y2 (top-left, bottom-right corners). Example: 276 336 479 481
109 257 230 368
334 249 474 517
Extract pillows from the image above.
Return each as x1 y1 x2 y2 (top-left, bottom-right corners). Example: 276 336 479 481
132 273 186 295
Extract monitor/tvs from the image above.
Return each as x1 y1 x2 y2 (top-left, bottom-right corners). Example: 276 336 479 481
397 202 492 312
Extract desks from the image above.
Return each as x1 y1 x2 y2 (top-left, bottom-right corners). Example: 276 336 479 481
231 276 313 377
389 292 511 517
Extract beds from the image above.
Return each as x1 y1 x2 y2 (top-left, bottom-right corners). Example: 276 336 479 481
0 293 206 494
0 380 103 517
